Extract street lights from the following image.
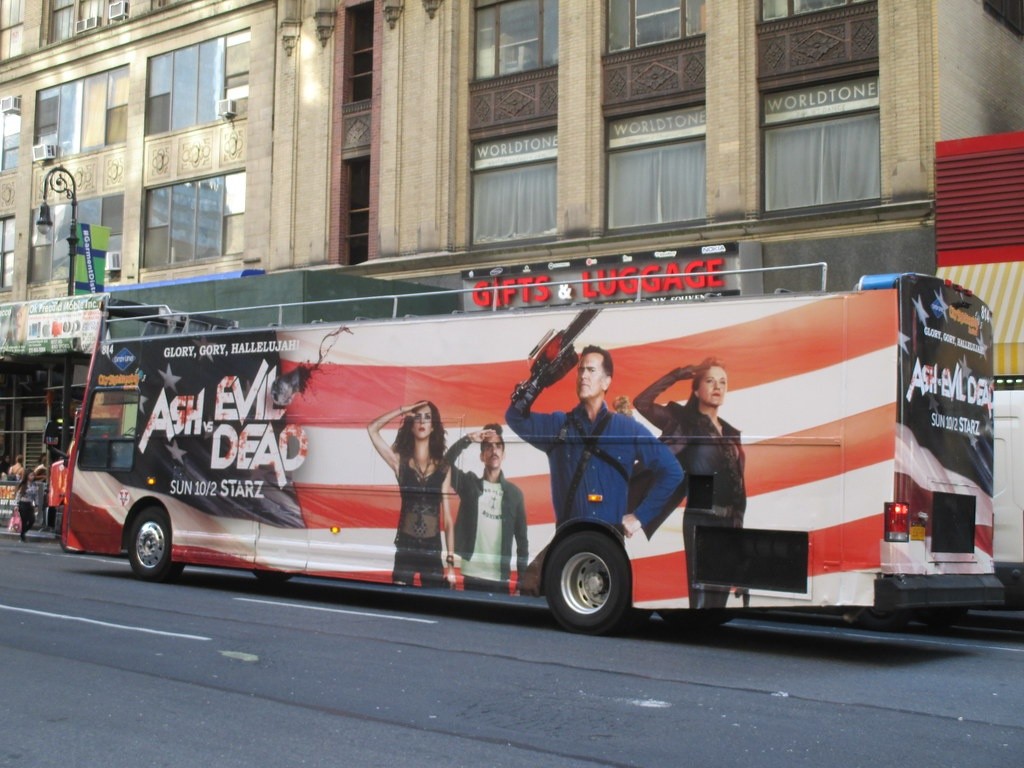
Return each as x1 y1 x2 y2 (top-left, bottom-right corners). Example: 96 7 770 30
36 167 80 534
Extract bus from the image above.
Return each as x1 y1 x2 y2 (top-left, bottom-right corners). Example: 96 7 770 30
61 261 1006 635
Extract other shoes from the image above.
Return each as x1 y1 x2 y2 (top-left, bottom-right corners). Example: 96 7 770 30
18 534 27 544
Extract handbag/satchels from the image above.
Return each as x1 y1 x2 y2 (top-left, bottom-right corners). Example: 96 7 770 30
8 509 22 532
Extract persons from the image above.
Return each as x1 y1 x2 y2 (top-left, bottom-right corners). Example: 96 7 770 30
367 346 753 612
1 454 58 544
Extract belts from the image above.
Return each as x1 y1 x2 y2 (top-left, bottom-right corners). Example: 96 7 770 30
684 504 746 520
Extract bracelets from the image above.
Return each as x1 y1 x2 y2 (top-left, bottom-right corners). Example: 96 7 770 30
446 555 455 566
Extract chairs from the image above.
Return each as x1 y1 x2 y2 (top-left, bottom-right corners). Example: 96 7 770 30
140 289 796 336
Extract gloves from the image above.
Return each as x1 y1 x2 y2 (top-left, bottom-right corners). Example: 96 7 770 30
671 365 709 380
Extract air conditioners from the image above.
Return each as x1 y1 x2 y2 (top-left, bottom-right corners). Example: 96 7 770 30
1 96 21 113
75 16 102 34
105 251 121 271
218 99 235 116
109 1 129 20
33 144 57 161
